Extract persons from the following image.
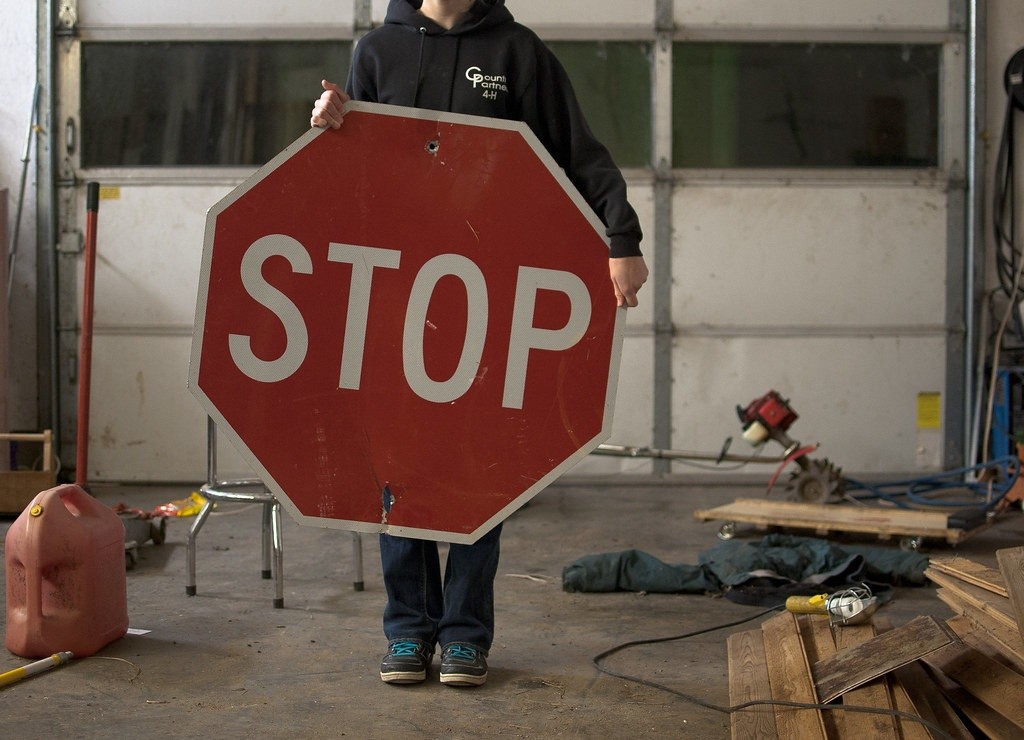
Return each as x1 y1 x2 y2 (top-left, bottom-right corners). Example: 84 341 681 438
309 0 651 691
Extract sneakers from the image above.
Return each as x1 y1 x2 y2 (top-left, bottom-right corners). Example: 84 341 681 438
440 641 489 687
379 637 435 683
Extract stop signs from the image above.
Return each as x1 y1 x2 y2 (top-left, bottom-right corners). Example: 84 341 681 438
190 95 657 544
